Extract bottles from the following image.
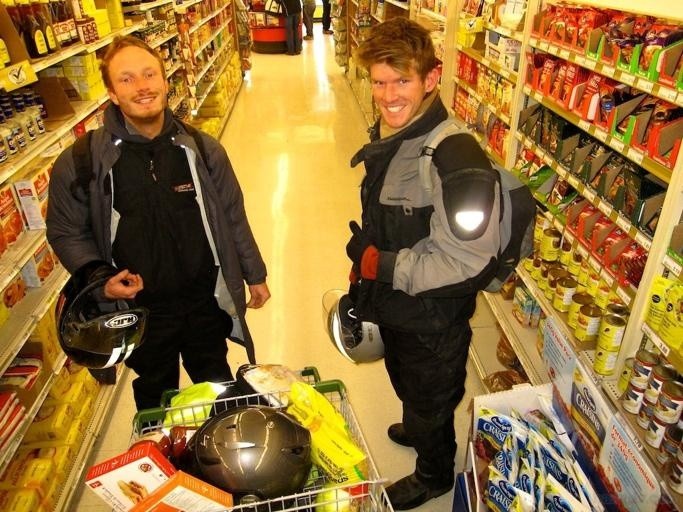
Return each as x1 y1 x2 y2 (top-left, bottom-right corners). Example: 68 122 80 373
0 0 79 71
0 86 48 165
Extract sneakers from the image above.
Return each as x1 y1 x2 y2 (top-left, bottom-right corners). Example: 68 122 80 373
381 459 453 509
387 422 415 448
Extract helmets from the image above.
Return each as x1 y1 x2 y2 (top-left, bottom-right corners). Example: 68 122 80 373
323 285 386 364
55 261 147 368
182 405 312 510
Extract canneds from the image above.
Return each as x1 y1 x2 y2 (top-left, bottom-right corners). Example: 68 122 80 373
519 211 683 496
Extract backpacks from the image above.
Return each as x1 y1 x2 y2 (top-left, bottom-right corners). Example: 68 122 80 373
420 120 534 293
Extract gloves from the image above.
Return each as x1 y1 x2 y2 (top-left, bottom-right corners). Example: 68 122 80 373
347 221 378 281
350 262 358 283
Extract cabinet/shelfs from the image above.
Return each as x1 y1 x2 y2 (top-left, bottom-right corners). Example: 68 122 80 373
0 1 254 512
329 0 683 512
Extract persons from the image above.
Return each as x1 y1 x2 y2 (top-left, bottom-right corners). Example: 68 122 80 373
301 2 317 41
274 0 300 54
44 34 270 427
320 1 333 36
344 17 499 510
